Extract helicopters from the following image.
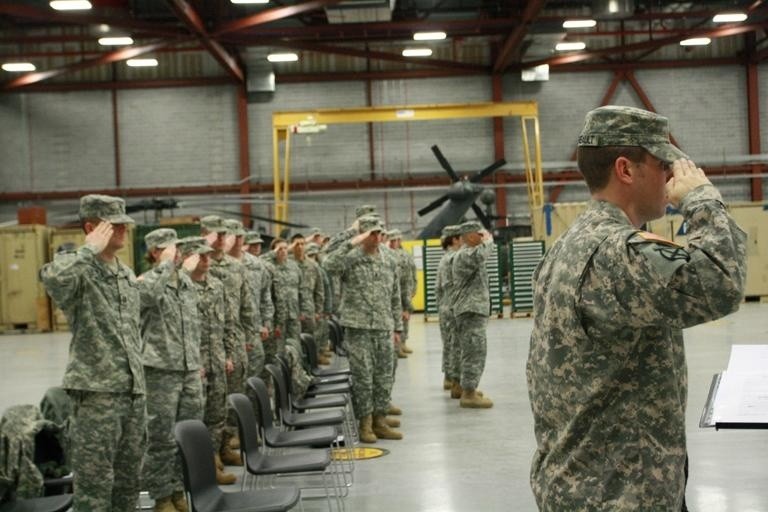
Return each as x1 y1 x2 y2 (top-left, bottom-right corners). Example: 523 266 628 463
0 144 507 243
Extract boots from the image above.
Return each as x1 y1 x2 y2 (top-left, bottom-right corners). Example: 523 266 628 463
155 492 191 512
215 438 244 486
317 345 332 365
397 340 412 358
360 404 403 442
443 378 492 407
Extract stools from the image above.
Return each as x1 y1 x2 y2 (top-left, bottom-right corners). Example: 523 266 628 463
1 489 77 511
39 463 80 493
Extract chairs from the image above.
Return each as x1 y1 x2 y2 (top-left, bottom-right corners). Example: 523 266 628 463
326 314 349 366
285 333 361 444
265 365 355 474
175 418 305 512
276 354 357 451
229 394 341 512
246 377 349 498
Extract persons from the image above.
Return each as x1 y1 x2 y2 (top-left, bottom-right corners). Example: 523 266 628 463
434 221 493 409
525 103 749 512
36 194 344 512
321 204 415 443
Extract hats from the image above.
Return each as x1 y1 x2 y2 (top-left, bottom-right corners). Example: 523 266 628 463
79 195 133 223
440 221 484 243
579 104 690 164
177 236 215 254
354 205 385 234
145 228 182 254
200 216 264 244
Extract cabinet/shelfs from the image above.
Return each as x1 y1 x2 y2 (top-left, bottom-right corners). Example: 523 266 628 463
17 206 47 226
